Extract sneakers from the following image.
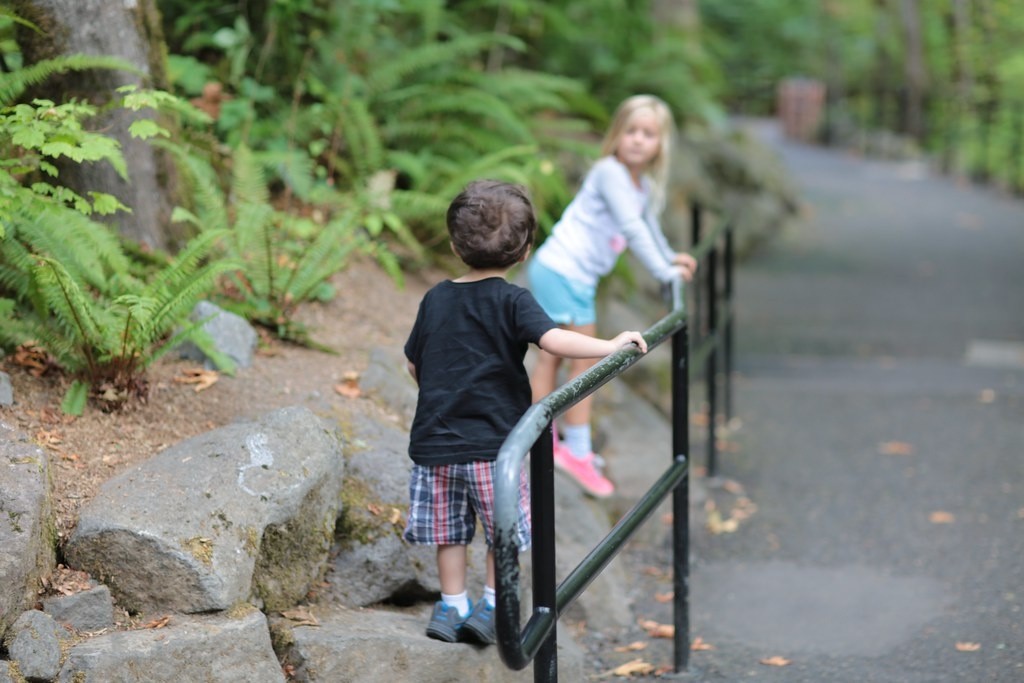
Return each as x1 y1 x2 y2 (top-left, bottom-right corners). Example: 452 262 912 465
427 597 475 643
462 599 497 645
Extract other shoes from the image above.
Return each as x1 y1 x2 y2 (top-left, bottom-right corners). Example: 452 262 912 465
555 446 614 498
553 421 559 459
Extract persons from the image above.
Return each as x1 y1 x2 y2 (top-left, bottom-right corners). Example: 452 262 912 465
529 94 697 498
404 179 648 645
